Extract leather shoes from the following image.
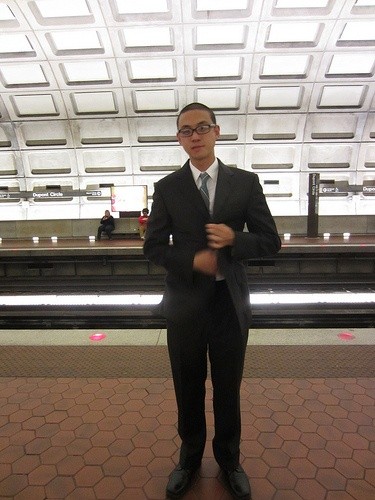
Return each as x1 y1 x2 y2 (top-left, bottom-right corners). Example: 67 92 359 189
221 461 252 500
165 462 202 500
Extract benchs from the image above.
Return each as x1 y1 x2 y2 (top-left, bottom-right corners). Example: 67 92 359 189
98 218 145 240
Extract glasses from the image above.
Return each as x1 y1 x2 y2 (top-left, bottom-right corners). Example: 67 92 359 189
177 123 216 139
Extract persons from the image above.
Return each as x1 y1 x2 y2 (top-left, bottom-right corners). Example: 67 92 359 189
139 208 149 239
142 103 285 498
96 209 115 240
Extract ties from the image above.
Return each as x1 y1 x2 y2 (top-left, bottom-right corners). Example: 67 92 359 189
196 172 211 209
